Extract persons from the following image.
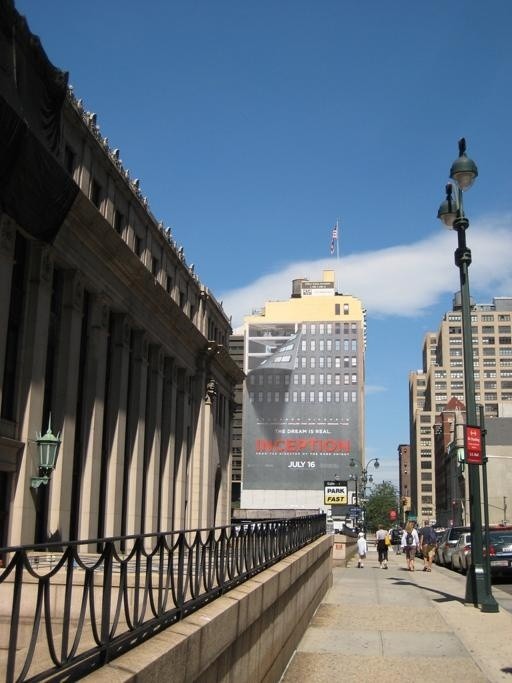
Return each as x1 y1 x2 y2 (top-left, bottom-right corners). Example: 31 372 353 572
400 522 420 571
375 524 390 569
388 523 404 555
356 532 367 568
419 520 438 572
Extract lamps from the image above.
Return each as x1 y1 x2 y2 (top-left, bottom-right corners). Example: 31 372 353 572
29 410 62 488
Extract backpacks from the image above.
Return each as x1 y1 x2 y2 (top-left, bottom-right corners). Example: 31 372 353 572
407 532 414 545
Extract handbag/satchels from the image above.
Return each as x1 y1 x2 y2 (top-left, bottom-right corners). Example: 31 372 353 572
384 535 391 546
429 534 433 546
360 554 366 559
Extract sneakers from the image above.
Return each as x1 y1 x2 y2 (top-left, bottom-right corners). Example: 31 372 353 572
358 562 431 572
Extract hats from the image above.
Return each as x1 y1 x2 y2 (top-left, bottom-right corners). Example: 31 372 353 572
359 532 365 536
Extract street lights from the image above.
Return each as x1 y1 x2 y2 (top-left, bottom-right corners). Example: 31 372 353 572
348 458 379 540
438 138 500 612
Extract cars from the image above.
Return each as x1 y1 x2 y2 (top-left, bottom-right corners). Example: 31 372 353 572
435 526 512 575
